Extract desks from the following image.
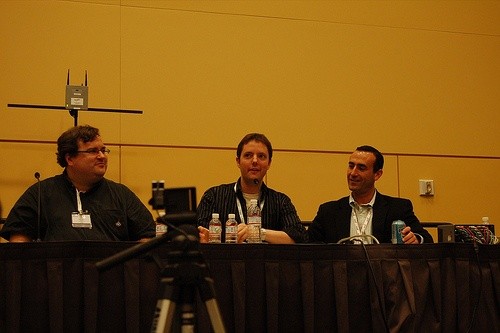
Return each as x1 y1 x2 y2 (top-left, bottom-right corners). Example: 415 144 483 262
0 242 500 333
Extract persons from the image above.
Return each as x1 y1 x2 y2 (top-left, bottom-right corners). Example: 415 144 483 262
0 124 156 242
196 133 308 243
307 145 434 244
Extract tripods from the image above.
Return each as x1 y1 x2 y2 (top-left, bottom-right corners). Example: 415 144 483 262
96 254 225 333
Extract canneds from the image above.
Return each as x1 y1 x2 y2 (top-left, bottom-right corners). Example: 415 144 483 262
391 220 406 244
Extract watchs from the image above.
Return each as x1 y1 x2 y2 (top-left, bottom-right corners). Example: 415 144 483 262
261 228 266 241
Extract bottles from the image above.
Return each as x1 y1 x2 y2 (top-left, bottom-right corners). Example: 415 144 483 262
226 214 238 244
155 212 167 236
482 216 489 224
247 200 262 243
209 213 221 244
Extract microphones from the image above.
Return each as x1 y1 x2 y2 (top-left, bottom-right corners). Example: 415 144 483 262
253 178 261 241
35 172 40 239
